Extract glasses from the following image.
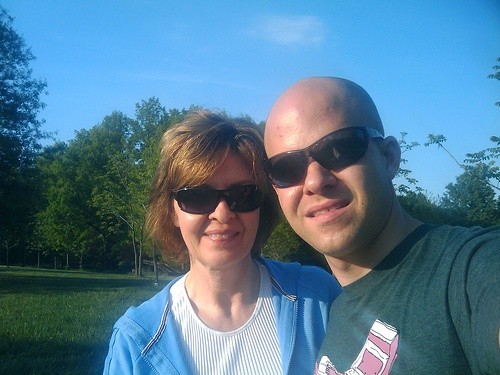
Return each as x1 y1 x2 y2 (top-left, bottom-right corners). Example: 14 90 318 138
171 181 265 216
261 124 385 190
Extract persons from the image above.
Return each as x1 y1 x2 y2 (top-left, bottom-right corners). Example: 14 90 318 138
263 76 500 375
101 106 344 375
